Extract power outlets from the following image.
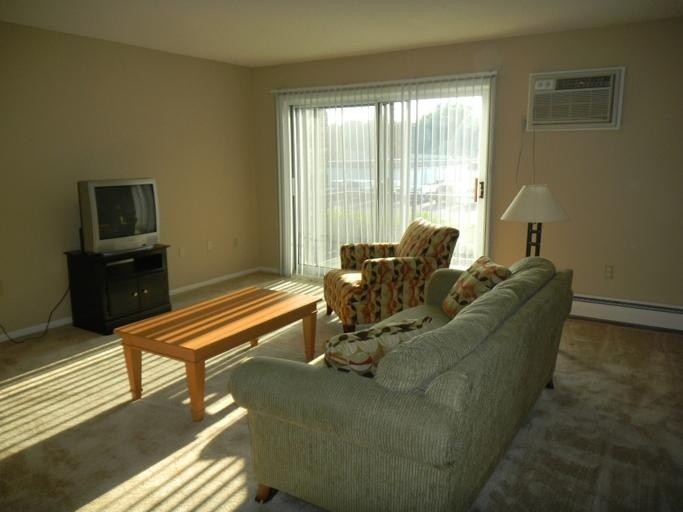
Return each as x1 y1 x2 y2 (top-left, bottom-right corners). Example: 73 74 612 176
604 266 613 279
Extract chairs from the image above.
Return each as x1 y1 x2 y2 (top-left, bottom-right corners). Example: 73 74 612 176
323 217 460 332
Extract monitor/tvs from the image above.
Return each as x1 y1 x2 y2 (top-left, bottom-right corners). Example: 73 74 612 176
77 176 160 257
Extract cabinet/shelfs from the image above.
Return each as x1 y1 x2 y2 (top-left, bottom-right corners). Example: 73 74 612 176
63 243 173 336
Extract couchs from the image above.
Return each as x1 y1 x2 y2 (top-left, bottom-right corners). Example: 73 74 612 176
228 252 571 511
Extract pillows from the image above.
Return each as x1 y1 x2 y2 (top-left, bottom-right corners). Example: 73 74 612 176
441 253 512 320
323 314 433 373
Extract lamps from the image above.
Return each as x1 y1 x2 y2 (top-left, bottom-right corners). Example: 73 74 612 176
499 183 572 257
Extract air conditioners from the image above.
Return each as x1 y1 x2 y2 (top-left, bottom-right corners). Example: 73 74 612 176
523 64 626 132
